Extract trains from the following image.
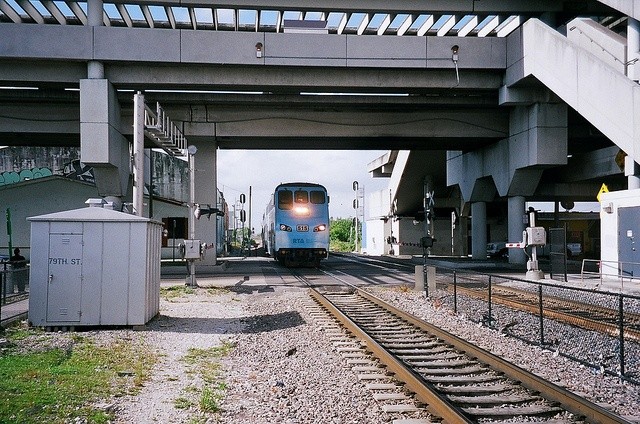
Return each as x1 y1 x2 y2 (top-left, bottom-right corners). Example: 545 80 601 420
261 182 330 267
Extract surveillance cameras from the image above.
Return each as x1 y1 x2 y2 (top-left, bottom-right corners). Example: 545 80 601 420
451 45 459 54
255 42 262 50
